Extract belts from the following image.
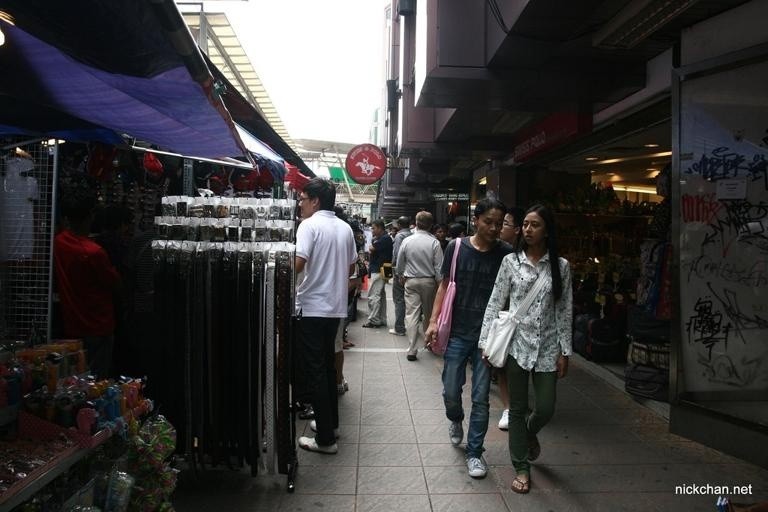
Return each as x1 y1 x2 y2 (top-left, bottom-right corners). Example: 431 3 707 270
147 201 291 477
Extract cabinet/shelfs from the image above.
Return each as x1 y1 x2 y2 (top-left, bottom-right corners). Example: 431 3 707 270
0 364 164 506
536 192 668 371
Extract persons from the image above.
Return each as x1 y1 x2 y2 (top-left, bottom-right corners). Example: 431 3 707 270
422 197 516 480
478 204 573 495
54 185 180 417
289 178 359 456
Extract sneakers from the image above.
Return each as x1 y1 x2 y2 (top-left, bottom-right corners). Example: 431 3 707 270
498 409 508 430
297 381 348 419
362 323 379 328
408 355 417 360
389 329 404 336
466 457 487 478
310 419 339 437
298 437 338 453
449 421 463 446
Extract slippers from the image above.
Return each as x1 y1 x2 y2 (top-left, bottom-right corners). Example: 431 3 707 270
528 437 540 461
511 477 529 493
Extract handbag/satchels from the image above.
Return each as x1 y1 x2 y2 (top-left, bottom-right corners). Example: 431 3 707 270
484 310 520 368
378 262 393 280
431 281 457 357
624 334 670 402
634 261 680 321
